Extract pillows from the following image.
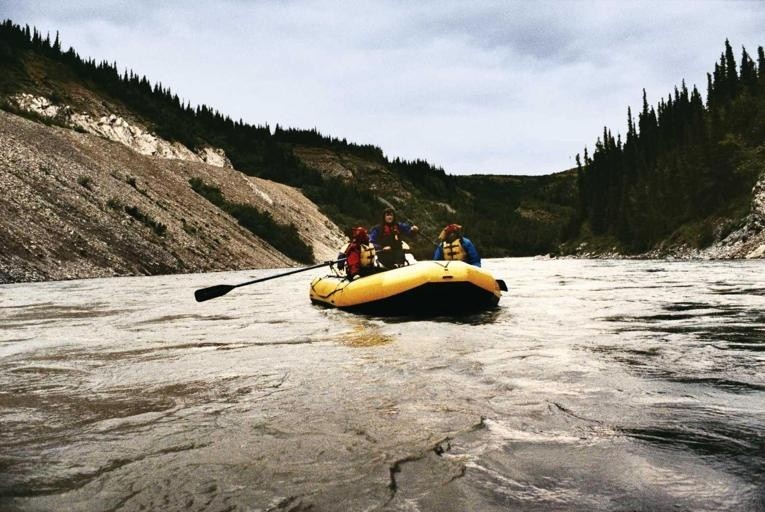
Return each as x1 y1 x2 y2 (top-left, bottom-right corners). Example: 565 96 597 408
350 226 368 245
444 225 460 234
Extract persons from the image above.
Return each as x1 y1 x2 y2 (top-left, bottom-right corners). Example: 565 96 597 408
369 208 420 270
432 224 482 267
346 224 387 281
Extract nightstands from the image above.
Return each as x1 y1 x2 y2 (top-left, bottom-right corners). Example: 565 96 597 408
416 230 508 291
194 250 386 302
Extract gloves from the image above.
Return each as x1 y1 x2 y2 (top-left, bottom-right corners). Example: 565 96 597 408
310 261 501 310
